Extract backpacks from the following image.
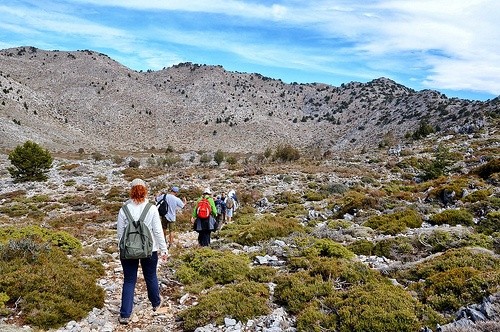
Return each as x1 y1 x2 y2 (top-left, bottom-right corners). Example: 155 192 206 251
214 201 223 216
119 203 153 259
196 196 212 218
225 197 233 209
156 194 168 216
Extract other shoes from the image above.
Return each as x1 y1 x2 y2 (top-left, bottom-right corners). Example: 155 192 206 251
153 295 163 312
224 220 227 225
118 316 128 324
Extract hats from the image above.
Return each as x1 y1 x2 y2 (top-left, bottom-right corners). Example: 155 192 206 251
171 187 179 193
131 178 147 188
217 195 221 198
202 188 212 195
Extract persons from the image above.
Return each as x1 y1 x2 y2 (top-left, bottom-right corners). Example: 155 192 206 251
158 187 238 247
117 179 169 324
